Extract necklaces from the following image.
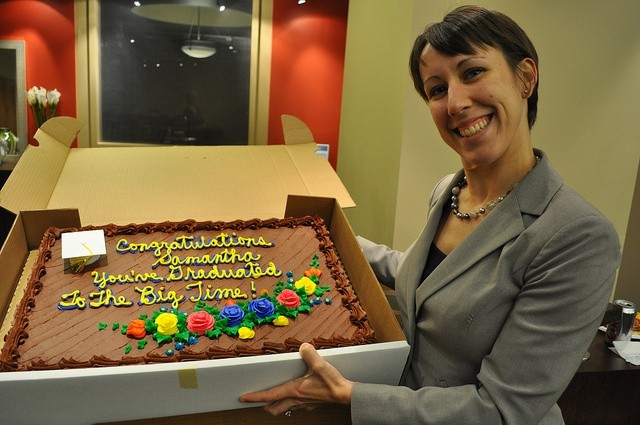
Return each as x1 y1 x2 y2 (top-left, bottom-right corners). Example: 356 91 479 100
450 155 541 219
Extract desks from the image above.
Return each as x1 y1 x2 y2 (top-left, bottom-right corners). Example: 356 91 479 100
557 327 640 425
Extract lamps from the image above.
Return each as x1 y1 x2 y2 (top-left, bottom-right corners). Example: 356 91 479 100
127 1 252 59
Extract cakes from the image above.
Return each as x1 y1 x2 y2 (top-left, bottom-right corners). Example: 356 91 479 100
0 215 373 374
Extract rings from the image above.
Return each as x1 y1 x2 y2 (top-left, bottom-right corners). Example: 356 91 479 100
285 410 296 417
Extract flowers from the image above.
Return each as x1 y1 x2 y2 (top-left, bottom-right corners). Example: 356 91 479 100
26 86 61 128
98 255 331 353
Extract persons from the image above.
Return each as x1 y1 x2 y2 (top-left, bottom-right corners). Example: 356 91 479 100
184 90 208 128
239 5 620 424
